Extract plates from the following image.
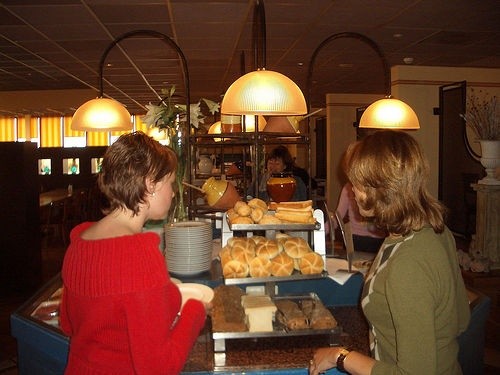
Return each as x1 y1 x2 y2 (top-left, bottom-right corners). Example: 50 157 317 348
146 220 213 277
28 288 62 327
176 282 214 314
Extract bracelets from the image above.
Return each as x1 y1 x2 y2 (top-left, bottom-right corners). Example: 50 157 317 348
336 347 356 373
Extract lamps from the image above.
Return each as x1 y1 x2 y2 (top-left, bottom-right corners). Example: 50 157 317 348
71 31 192 222
211 36 419 200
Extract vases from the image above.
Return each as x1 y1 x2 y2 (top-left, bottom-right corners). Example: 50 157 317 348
478 138 500 186
167 125 192 223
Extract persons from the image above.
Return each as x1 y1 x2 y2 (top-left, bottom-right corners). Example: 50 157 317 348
323 182 386 254
246 145 318 244
309 131 472 375
58 130 214 375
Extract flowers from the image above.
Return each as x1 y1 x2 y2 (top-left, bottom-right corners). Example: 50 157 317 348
462 89 500 140
143 89 219 136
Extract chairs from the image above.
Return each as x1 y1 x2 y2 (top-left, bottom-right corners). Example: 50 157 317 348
44 184 106 247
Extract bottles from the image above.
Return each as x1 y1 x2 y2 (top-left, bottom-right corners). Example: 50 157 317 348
266 173 297 203
197 154 242 211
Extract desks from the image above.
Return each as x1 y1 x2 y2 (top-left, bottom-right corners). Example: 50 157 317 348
40 187 85 207
469 182 500 273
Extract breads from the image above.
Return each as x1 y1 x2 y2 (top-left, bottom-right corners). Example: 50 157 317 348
210 285 337 332
219 233 324 278
226 198 316 225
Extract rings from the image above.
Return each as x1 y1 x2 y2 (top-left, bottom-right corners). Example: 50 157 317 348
310 360 314 365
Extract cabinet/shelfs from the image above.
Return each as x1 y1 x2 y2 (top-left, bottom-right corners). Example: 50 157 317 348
211 212 330 355
